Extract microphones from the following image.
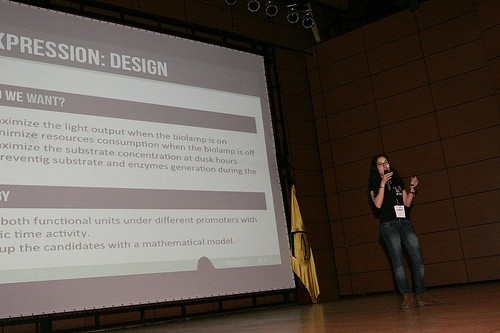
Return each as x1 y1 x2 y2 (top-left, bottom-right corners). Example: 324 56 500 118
384 170 391 191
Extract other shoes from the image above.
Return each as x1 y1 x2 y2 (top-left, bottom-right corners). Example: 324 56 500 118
416 293 443 305
401 292 416 308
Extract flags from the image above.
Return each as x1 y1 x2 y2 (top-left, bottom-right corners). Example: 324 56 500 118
290 187 320 303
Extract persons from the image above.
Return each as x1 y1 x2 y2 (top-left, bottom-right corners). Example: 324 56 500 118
369 154 441 309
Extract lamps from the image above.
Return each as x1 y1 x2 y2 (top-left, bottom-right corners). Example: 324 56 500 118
264 0 278 18
247 0 261 13
225 0 238 6
286 0 300 24
300 0 314 28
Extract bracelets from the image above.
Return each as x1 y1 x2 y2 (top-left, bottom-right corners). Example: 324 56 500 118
410 190 415 196
379 187 385 188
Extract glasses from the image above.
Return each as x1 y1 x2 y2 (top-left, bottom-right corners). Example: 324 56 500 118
377 160 389 167
